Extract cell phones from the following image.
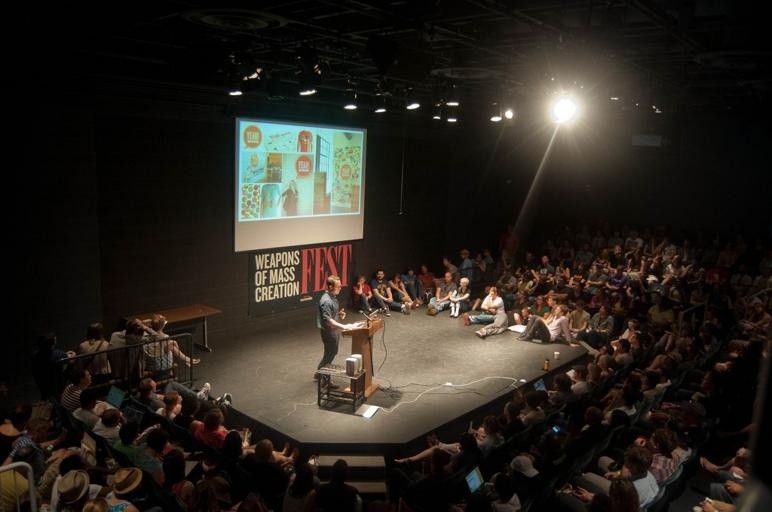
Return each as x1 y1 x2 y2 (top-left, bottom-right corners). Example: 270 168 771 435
552 427 559 433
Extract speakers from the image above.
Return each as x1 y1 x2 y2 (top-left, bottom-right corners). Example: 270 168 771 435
350 353 363 371
345 358 358 376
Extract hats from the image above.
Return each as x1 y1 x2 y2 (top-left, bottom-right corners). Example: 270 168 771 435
57 469 90 503
112 467 142 494
0 423 26 436
646 275 658 281
510 456 539 477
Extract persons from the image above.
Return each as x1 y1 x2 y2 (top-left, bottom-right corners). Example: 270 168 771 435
3 309 367 512
352 219 771 512
282 179 300 215
313 275 356 388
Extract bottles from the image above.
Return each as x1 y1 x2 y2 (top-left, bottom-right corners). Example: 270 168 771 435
544 359 549 371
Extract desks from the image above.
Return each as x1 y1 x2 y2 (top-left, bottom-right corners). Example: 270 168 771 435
123 301 222 353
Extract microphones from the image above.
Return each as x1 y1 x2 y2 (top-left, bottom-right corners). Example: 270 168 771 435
357 309 373 322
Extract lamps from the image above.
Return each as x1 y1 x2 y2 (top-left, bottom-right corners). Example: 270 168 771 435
230 67 516 124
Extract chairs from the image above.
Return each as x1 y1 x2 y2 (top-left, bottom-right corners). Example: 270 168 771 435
50 392 283 512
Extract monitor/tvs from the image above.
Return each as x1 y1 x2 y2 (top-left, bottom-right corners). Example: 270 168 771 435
464 466 485 495
533 378 547 392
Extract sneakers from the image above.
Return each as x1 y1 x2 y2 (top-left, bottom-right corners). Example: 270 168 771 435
475 328 487 337
329 381 340 388
283 442 299 463
516 334 532 340
368 301 419 318
427 303 437 316
449 312 470 325
186 358 201 368
198 383 210 399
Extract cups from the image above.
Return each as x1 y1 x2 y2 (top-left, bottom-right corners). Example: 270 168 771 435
554 351 560 360
